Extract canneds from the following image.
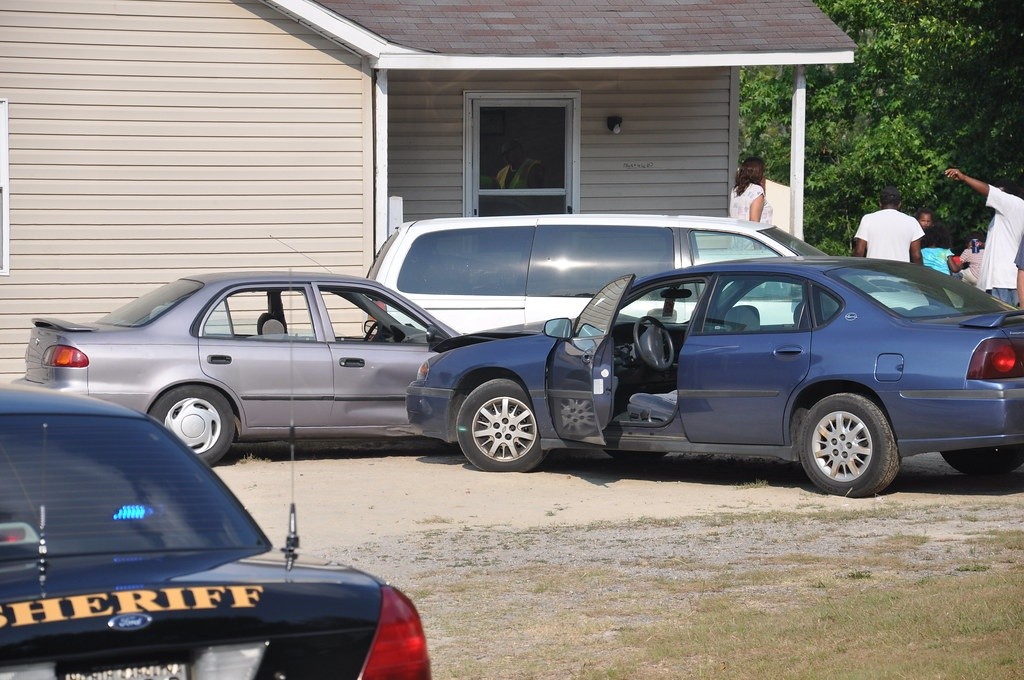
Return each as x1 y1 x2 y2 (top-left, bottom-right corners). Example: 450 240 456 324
972 239 979 253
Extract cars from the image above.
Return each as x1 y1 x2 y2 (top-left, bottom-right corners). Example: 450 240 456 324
0 388 433 679
406 252 1023 499
13 268 466 467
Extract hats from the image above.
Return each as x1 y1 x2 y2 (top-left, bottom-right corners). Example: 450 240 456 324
880 185 900 201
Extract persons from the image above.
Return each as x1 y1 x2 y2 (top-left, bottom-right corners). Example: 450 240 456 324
729 156 767 222
853 185 925 264
915 167 1024 315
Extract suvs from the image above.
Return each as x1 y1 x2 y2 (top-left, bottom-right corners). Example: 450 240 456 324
363 211 938 344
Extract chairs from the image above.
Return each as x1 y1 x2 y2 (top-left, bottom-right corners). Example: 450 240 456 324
257 313 285 341
627 305 761 422
793 302 809 329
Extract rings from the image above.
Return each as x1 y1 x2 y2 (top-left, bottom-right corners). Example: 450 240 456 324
956 171 958 175
